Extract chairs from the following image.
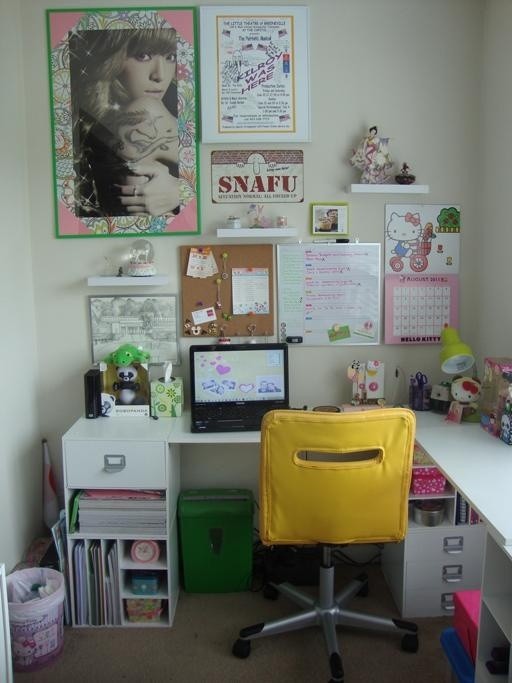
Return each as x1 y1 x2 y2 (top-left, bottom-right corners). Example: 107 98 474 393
228 408 421 682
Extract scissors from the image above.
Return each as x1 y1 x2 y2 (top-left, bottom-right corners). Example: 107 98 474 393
416 372 429 388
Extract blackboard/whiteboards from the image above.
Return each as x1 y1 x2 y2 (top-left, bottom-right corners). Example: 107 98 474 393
276 243 380 346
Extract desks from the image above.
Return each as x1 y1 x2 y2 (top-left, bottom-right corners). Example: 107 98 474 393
61 402 512 683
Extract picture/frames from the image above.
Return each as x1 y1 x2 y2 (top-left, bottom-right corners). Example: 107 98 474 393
46 6 201 239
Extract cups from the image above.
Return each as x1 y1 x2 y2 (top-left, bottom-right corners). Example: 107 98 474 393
414 386 431 411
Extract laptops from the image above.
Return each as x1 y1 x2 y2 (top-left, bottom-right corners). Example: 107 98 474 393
190 342 292 433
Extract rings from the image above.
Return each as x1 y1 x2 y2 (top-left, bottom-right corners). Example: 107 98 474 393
133 185 137 197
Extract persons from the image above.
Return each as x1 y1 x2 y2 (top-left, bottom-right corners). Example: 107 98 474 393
73 30 180 217
350 126 392 184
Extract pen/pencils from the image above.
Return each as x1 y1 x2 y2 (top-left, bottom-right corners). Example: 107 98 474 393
313 238 350 243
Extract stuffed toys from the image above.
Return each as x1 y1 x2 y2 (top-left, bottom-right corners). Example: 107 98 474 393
103 344 151 367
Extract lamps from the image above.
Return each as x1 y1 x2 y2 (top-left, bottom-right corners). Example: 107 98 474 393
441 328 491 423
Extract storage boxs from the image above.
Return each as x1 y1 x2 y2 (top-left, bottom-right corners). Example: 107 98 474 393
437 588 481 682
175 485 257 595
